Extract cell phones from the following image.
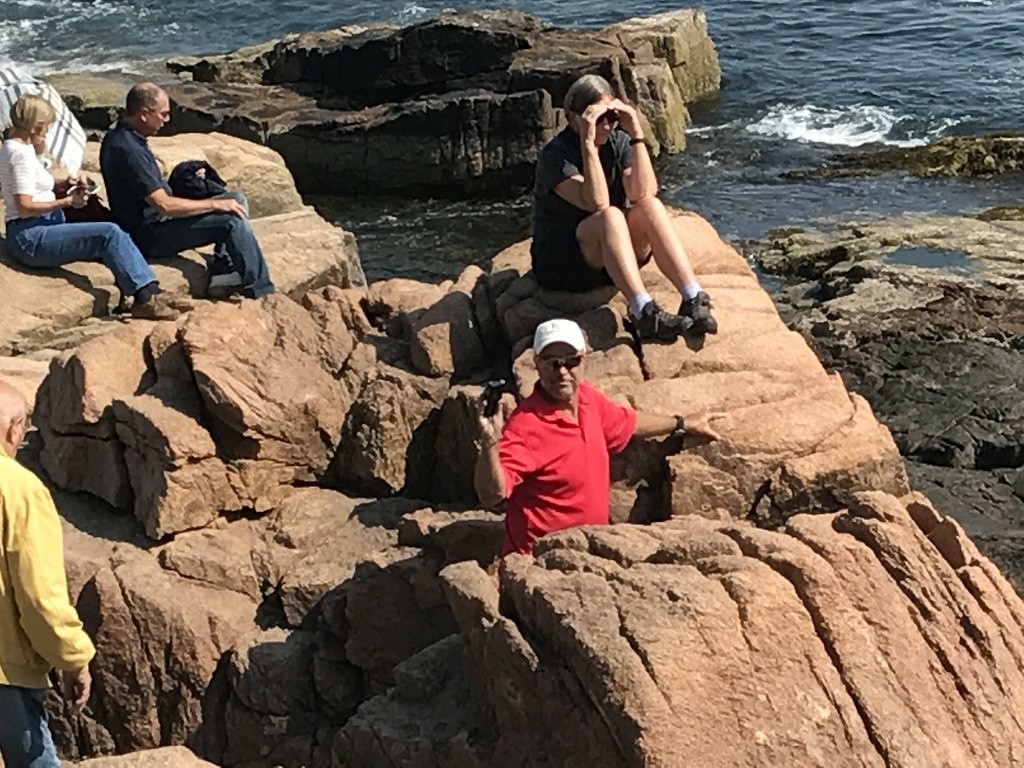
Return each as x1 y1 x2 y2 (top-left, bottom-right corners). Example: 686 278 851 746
482 379 506 417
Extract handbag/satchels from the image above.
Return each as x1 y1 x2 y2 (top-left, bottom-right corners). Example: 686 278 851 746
168 159 228 198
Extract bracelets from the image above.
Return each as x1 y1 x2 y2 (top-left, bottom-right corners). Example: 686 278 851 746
629 138 647 146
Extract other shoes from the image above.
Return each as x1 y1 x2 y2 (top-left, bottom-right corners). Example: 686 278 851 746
157 290 195 311
130 293 181 320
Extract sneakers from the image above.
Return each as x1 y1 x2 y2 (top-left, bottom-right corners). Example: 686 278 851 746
628 300 695 343
677 291 719 334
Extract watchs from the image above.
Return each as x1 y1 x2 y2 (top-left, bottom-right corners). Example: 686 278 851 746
671 415 686 437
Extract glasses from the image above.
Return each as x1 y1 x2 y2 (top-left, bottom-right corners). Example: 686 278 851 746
539 354 583 369
597 112 623 127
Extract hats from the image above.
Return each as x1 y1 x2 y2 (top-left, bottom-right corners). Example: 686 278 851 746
533 318 585 359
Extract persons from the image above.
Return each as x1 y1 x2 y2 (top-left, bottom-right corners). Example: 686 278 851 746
0 380 96 768
474 318 728 556
530 74 718 335
0 82 276 320
